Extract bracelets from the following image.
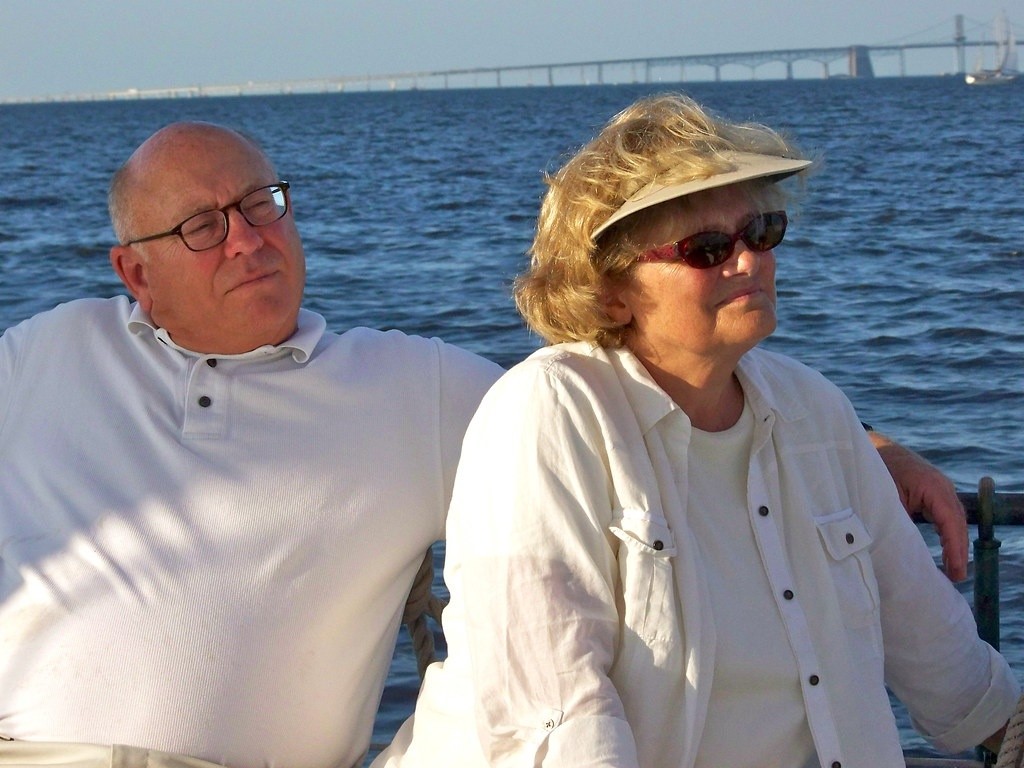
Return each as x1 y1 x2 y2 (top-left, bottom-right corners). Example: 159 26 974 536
860 421 874 433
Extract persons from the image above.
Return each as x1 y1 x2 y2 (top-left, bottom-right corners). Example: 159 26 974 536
369 90 1024 767
0 118 971 767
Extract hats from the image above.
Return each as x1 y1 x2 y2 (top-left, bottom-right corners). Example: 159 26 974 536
590 152 812 238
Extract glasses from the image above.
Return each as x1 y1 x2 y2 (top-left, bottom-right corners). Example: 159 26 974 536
127 181 291 252
632 210 789 269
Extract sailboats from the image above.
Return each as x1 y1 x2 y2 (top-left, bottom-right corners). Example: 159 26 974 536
965 7 1019 86
